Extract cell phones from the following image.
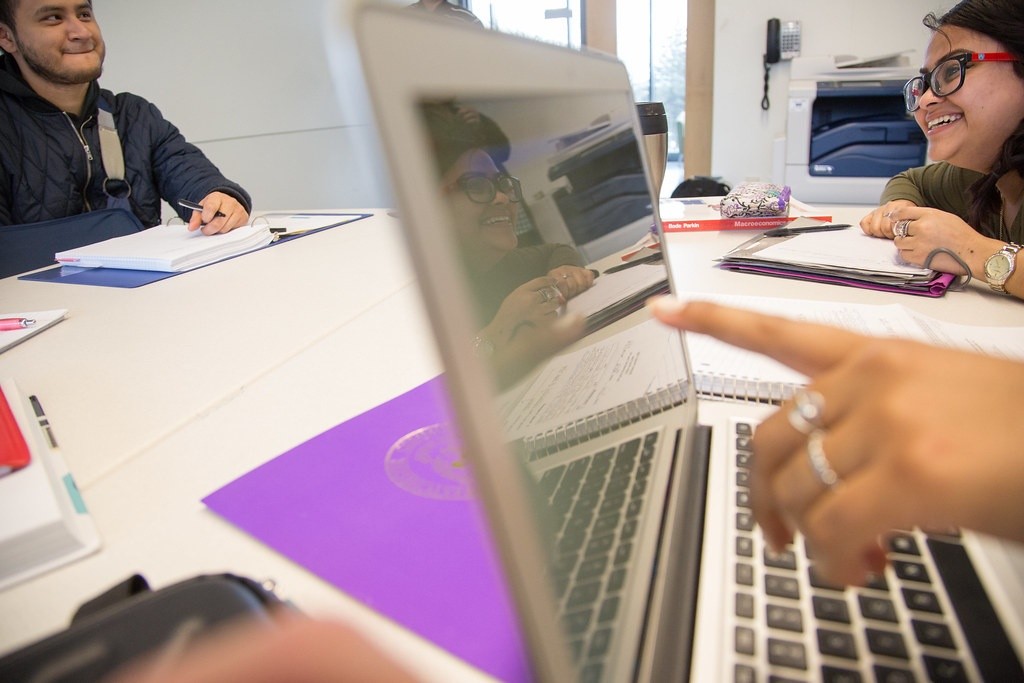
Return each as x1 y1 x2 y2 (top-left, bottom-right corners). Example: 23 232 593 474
0 510 102 591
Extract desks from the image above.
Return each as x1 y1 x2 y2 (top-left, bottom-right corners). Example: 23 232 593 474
0 207 1024 683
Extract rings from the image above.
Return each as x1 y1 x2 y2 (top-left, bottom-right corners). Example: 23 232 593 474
882 213 889 217
538 289 551 302
809 433 836 488
894 220 912 236
562 273 571 279
791 396 821 433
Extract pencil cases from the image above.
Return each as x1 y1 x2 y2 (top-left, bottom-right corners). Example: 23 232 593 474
721 178 791 215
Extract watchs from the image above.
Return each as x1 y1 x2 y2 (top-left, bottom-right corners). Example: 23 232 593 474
984 242 1024 295
468 334 494 361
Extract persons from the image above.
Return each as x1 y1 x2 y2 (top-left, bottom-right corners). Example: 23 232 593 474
859 0 1024 301
424 105 598 361
646 297 1024 585
0 0 252 237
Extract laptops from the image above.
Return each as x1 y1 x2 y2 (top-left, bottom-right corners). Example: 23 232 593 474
348 0 1024 683
529 401 687 683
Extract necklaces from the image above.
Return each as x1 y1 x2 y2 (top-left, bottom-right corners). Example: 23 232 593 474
999 208 1004 240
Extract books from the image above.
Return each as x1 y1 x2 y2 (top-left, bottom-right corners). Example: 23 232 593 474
0 375 99 594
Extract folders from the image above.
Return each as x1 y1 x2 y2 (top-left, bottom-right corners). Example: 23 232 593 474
16 213 374 288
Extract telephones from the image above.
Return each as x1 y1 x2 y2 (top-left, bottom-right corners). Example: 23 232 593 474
767 19 800 64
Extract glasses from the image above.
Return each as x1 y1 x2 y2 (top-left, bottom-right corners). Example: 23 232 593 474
440 175 522 203
904 52 1022 112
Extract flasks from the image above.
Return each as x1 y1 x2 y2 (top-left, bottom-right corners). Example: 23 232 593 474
636 101 669 211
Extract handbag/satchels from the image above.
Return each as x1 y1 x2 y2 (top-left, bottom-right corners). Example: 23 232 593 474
0 95 144 279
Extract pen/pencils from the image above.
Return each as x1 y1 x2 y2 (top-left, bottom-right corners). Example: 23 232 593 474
178 197 224 219
763 223 853 236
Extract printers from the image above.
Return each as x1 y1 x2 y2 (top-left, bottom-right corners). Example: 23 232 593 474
543 119 657 265
785 46 940 205
